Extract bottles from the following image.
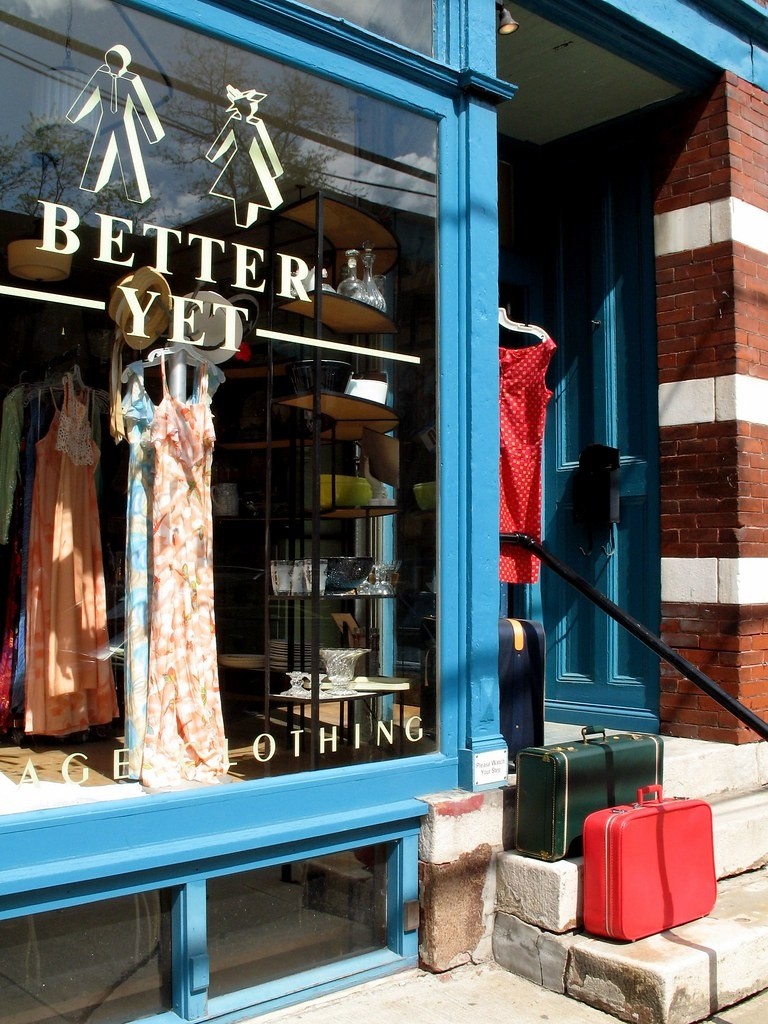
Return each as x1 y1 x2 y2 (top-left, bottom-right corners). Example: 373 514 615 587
322 239 387 312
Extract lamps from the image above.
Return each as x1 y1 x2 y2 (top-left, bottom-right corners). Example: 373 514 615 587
497 2 521 35
7 239 72 283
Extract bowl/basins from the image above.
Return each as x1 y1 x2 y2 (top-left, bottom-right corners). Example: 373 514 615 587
285 359 353 397
346 378 388 404
322 556 375 594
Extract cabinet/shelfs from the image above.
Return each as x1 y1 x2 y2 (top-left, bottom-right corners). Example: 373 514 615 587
211 363 347 741
265 191 405 761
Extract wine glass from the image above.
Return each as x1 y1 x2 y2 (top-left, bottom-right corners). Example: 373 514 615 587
319 648 372 696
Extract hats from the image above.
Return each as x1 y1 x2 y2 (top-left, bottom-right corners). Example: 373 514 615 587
109 264 172 350
178 291 244 366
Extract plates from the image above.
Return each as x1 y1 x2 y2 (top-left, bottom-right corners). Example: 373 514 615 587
219 638 311 673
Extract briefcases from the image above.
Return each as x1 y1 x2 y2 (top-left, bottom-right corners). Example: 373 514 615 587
583 784 716 941
420 617 546 770
515 725 664 860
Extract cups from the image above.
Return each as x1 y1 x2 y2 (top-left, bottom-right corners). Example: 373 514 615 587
269 559 327 596
211 482 239 516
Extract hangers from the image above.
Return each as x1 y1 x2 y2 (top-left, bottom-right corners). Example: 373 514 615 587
120 336 209 384
8 363 109 414
147 316 227 384
499 305 549 341
